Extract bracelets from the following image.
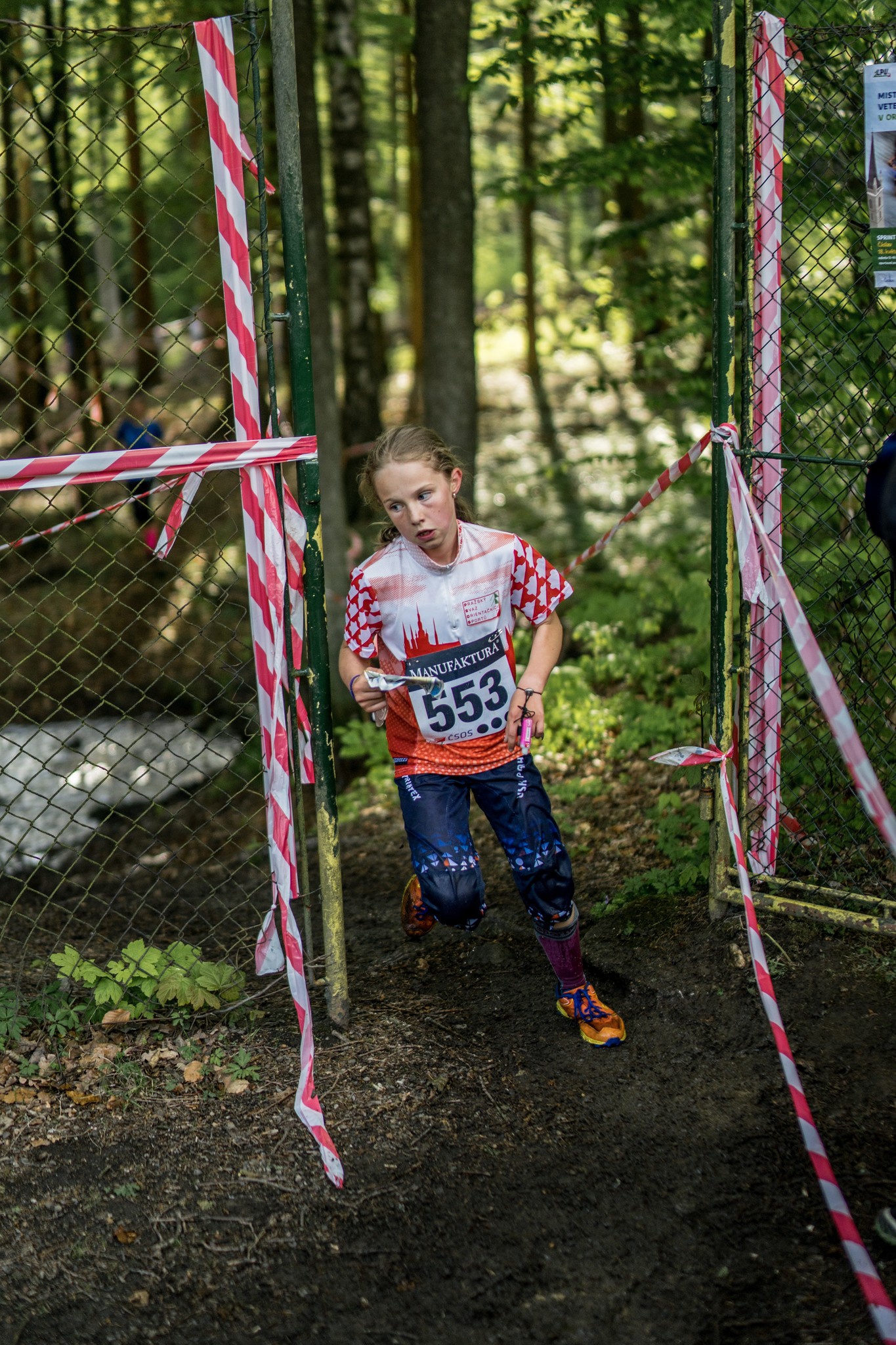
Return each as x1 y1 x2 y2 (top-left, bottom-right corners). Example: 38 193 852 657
515 686 542 735
350 670 363 699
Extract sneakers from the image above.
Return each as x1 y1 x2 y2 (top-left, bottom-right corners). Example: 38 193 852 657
554 975 630 1047
400 874 438 940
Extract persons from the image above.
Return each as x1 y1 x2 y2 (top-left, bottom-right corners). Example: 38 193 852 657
336 422 626 1047
115 398 163 529
